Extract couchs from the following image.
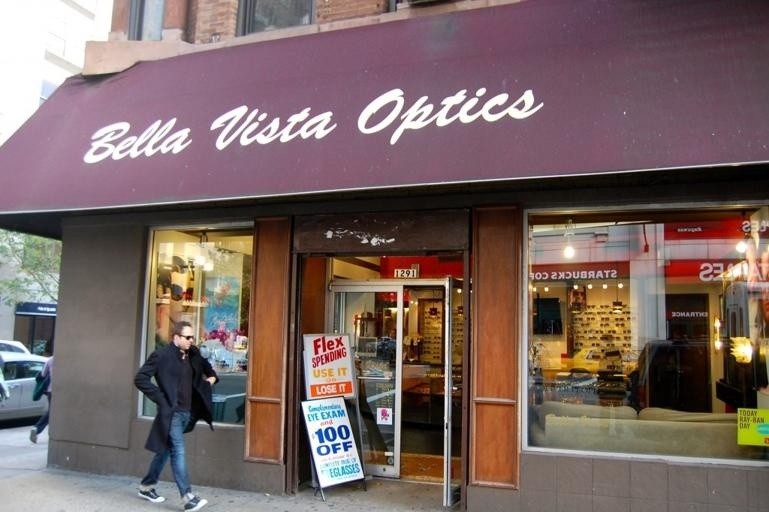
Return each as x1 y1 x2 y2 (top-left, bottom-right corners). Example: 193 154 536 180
530 399 755 464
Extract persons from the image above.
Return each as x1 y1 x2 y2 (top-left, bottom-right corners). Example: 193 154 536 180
636 322 694 411
594 333 627 441
29 336 54 444
743 224 769 395
570 285 585 305
131 319 219 511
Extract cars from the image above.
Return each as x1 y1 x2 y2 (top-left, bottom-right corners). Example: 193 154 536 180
0 340 51 424
200 338 248 371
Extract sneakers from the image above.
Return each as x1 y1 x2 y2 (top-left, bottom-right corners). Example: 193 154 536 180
139 488 165 503
30 427 38 443
184 495 208 512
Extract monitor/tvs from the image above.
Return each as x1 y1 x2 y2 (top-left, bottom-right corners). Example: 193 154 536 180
357 336 377 358
534 298 563 335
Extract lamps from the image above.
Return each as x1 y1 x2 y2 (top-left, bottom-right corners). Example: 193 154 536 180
735 230 751 257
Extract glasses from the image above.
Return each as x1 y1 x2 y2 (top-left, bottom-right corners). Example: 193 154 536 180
180 334 195 341
